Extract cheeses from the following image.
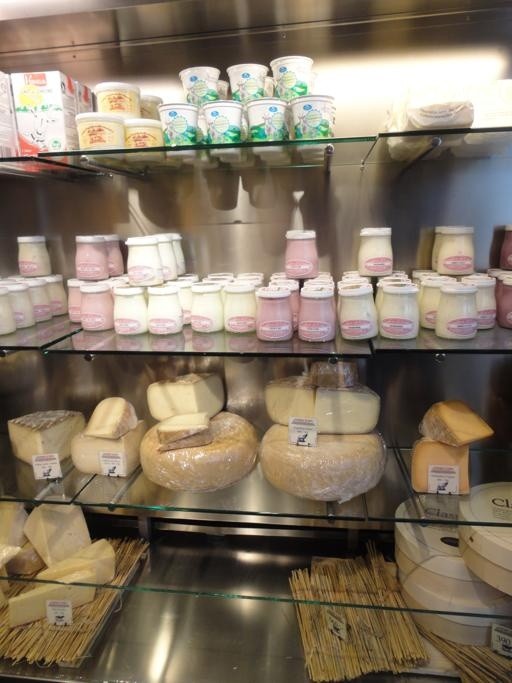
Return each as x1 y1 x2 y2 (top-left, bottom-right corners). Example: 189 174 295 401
6 408 86 468
0 492 119 629
260 360 389 506
138 371 258 494
410 399 495 495
71 395 146 479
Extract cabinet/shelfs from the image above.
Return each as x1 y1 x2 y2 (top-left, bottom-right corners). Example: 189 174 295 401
1 124 512 680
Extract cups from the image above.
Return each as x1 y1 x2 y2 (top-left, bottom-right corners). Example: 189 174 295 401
73 55 336 176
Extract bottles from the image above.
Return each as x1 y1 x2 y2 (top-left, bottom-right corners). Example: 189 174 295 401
356 227 395 279
76 235 125 284
18 238 52 277
500 226 512 272
282 231 319 278
69 269 512 343
125 232 186 285
0 271 68 336
431 227 476 277
